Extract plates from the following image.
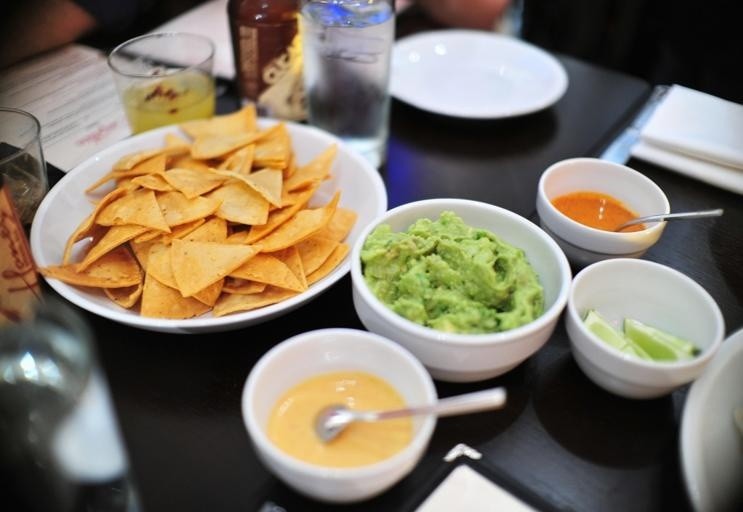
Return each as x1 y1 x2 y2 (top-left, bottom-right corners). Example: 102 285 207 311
389 30 572 127
28 112 390 338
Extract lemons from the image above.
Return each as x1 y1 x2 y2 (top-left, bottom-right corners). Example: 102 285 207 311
584 310 700 362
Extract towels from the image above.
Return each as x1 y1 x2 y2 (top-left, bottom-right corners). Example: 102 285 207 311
632 82 743 193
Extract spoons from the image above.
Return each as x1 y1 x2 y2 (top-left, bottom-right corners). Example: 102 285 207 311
309 384 508 448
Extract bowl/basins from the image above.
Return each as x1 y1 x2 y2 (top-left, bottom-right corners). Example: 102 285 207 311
350 194 574 386
674 324 743 510
565 257 727 403
535 155 671 268
237 325 442 509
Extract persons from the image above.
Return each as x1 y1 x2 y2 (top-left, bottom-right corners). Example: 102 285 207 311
1 1 210 73
395 1 512 40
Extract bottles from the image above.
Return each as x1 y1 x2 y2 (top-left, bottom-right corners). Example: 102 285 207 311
222 1 397 171
1 181 139 510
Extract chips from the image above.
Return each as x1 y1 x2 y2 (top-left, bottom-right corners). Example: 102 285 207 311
35 102 357 319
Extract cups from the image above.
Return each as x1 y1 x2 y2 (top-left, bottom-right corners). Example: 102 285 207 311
0 107 49 220
108 31 215 137
